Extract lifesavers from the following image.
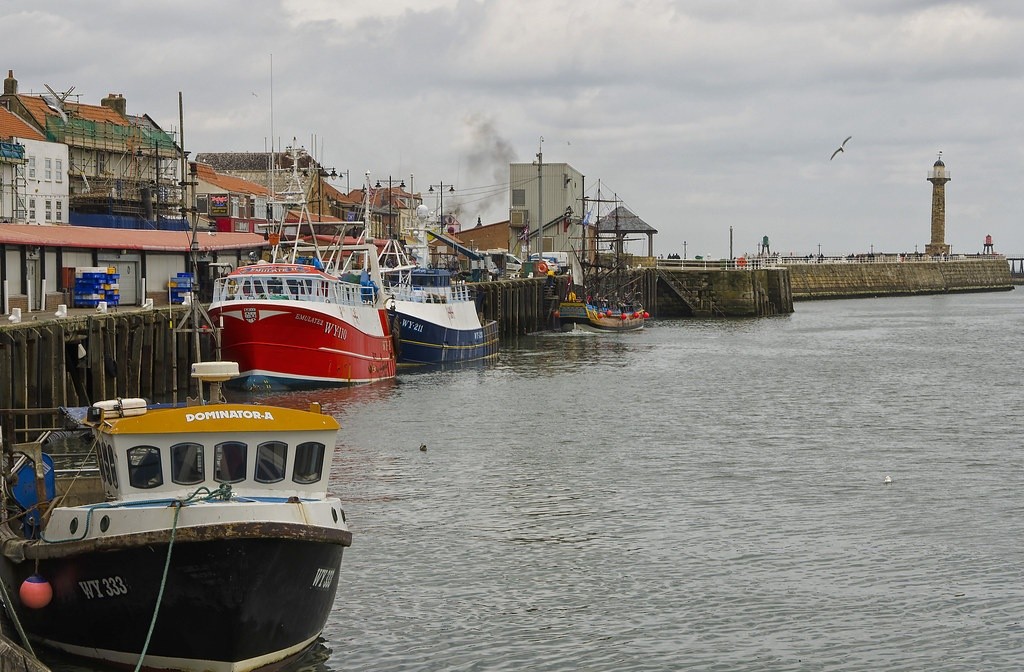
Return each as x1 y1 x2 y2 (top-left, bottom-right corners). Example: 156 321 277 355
738 257 746 267
538 263 547 272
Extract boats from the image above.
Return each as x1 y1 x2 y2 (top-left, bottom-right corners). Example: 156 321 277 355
0 361 353 672
385 205 500 365
551 176 649 333
208 137 396 393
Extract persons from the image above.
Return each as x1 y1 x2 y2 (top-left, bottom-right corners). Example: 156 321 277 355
667 253 680 266
914 251 923 261
805 253 824 263
934 251 950 261
564 291 576 302
977 252 980 258
848 253 875 263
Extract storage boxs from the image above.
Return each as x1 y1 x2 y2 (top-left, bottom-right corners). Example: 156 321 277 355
74 272 120 306
166 272 194 303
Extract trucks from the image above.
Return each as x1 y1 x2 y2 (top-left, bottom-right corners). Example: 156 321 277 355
475 251 569 274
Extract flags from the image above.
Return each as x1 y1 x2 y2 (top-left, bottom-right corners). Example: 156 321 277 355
518 228 529 240
582 211 590 224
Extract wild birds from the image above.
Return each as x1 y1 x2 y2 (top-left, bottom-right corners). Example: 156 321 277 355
830 136 852 160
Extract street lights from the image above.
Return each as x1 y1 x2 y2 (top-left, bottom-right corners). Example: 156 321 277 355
303 167 337 234
429 182 455 234
376 176 406 238
